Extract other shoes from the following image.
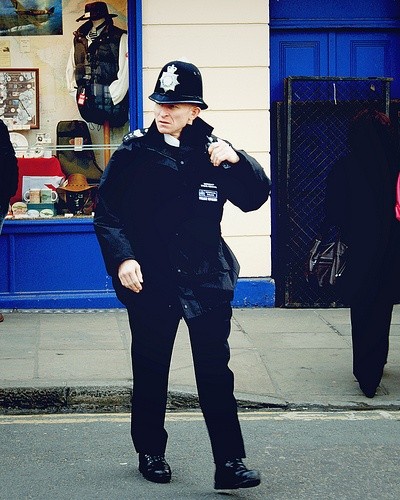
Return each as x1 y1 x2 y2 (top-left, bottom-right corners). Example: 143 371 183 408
358 383 377 399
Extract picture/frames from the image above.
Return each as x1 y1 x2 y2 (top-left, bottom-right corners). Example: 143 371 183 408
0 67 39 130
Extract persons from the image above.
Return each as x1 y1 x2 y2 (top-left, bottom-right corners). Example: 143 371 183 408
322 111 400 398
92 60 272 490
0 118 18 323
73 2 130 129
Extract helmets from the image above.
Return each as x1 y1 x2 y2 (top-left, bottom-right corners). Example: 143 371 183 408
148 61 208 110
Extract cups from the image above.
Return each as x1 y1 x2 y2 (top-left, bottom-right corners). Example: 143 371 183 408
23 189 40 203
36 133 45 143
3 118 13 130
44 151 53 158
41 189 58 203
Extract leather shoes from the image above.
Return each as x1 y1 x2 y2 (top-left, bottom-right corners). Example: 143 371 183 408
213 457 261 489
138 452 172 483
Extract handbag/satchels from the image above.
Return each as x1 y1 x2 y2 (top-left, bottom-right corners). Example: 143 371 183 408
303 225 353 288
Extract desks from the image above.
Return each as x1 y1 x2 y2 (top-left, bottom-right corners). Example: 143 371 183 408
7 154 66 206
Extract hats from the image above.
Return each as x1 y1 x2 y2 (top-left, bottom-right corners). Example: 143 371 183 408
76 2 118 22
56 173 96 202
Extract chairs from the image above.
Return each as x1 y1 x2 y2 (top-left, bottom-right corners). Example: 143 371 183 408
55 119 104 186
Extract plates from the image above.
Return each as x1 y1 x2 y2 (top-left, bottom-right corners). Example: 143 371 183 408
30 143 44 158
9 132 28 156
